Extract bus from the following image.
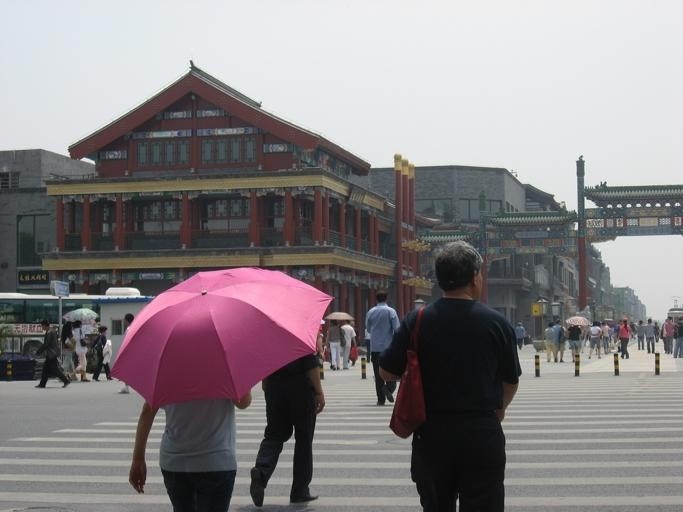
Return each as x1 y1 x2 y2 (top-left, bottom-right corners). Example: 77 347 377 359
0 291 101 360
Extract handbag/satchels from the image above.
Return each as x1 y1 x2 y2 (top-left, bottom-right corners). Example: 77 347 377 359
350 346 358 362
389 306 431 440
79 328 88 347
65 336 74 349
340 328 346 346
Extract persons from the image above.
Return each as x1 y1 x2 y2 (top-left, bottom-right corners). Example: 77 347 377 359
379 240 522 512
35 313 135 393
326 320 342 370
340 321 357 369
128 390 253 512
249 354 325 506
544 316 683 362
365 291 400 406
515 322 526 350
317 336 323 352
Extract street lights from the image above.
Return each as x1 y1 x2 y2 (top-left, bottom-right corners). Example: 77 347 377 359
539 297 549 337
550 300 560 325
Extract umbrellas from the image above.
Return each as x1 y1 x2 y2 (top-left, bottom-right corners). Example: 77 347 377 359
325 312 355 320
109 267 335 413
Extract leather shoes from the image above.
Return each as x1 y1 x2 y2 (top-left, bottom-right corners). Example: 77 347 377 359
382 384 394 402
35 384 45 388
249 467 268 507
377 401 384 406
289 493 318 502
62 380 70 388
